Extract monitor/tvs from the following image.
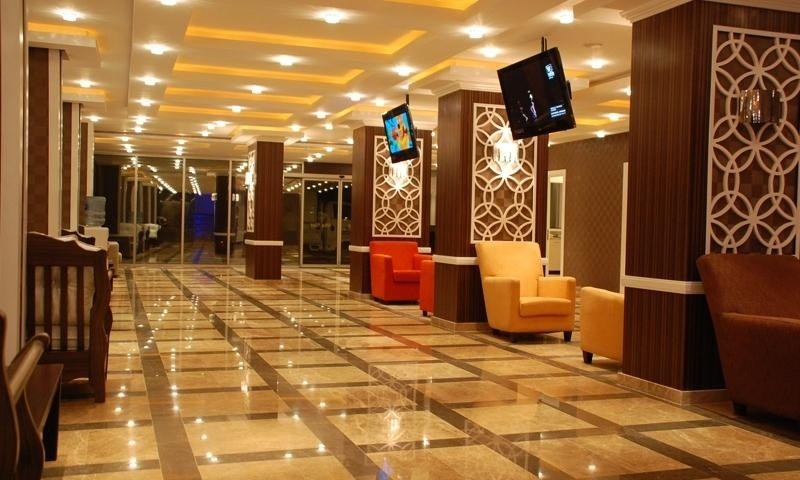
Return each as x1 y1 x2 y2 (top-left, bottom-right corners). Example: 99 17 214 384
497 47 576 141
381 103 419 163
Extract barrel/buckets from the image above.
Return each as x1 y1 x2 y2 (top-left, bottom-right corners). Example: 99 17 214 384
81 196 106 226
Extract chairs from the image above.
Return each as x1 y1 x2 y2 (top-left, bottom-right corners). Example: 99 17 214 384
474 239 577 344
119 222 161 258
368 241 435 317
28 225 114 404
695 252 800 426
579 285 624 366
